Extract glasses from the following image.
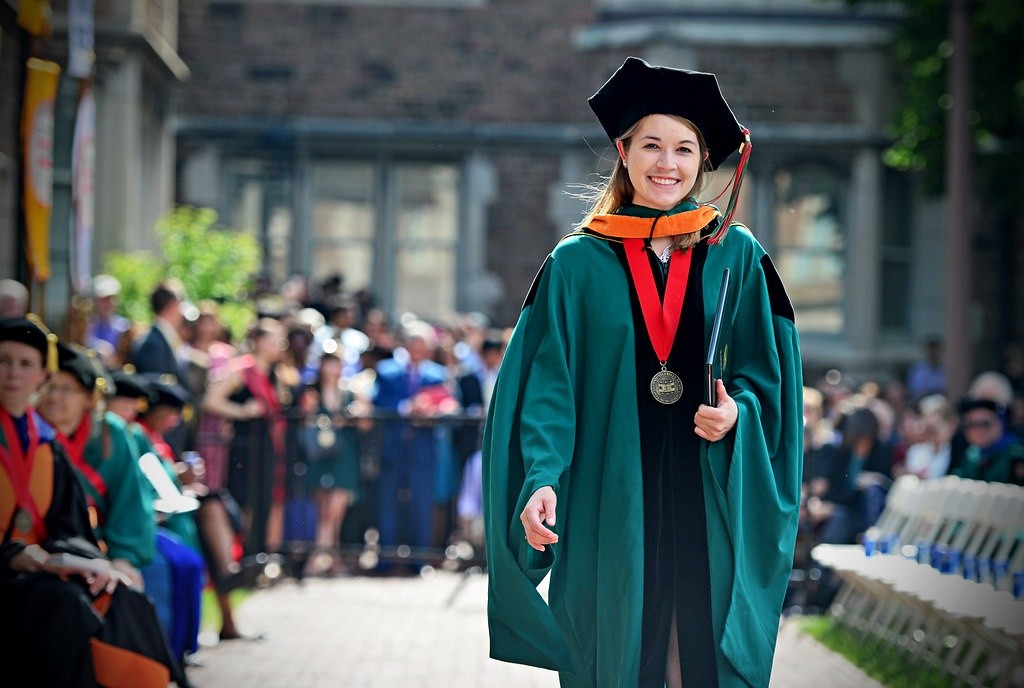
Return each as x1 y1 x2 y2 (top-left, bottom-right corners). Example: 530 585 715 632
958 418 1002 432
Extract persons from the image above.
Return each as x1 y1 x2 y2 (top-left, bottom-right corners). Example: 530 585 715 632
480 57 805 688
909 336 947 394
0 266 515 688
783 373 1024 620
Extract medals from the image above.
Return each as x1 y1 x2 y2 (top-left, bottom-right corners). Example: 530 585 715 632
650 371 683 404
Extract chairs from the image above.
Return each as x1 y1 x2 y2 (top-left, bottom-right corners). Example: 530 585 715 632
812 473 1024 688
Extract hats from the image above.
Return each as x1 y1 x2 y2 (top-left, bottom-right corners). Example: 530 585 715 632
108 368 195 407
587 57 749 246
955 392 1009 420
1 314 56 374
50 338 116 397
